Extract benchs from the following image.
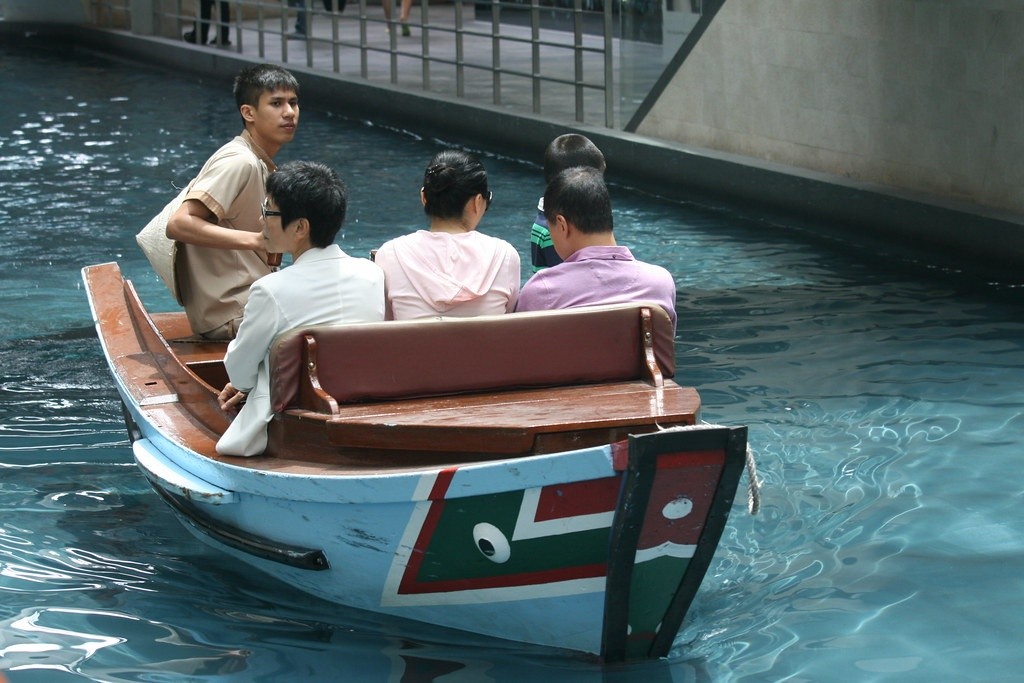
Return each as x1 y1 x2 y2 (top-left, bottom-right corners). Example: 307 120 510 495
269 301 676 413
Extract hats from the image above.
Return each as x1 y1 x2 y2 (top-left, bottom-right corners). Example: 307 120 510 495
136 176 197 306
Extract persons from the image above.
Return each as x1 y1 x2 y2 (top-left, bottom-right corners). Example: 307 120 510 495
531 132 608 274
166 63 299 343
514 166 678 336
376 152 522 322
214 162 386 458
183 0 412 48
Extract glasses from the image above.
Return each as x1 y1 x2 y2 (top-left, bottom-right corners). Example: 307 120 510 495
260 196 281 220
485 191 493 210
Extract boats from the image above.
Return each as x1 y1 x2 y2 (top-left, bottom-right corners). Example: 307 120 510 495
80 261 750 666
